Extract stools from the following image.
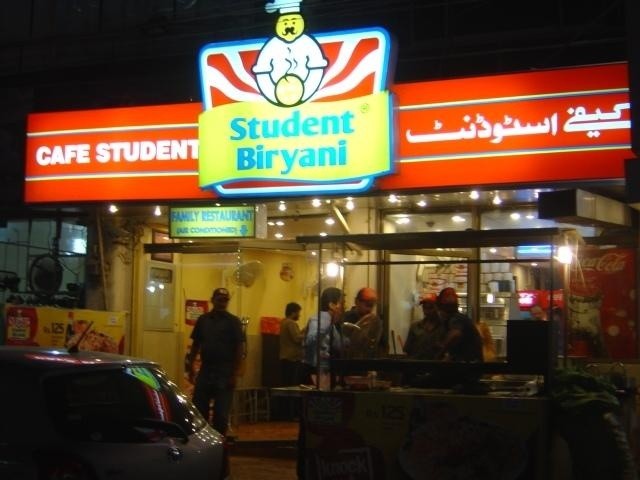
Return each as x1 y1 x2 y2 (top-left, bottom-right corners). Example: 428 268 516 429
233 387 270 424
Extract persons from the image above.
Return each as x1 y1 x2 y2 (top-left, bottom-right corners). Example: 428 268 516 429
301 287 347 389
334 287 385 385
278 302 315 386
434 287 484 389
186 288 247 437
529 303 547 320
402 293 447 385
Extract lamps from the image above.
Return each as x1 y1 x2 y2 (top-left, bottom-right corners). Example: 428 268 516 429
325 257 338 277
557 233 572 264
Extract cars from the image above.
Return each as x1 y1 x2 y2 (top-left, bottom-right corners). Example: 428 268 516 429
0 346 229 480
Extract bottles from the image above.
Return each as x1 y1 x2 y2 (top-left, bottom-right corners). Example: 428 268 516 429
319 346 331 391
367 372 376 390
562 284 604 359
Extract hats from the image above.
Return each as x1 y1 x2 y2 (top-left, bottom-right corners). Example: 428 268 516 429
434 288 458 305
357 288 377 302
419 294 437 304
213 288 231 300
